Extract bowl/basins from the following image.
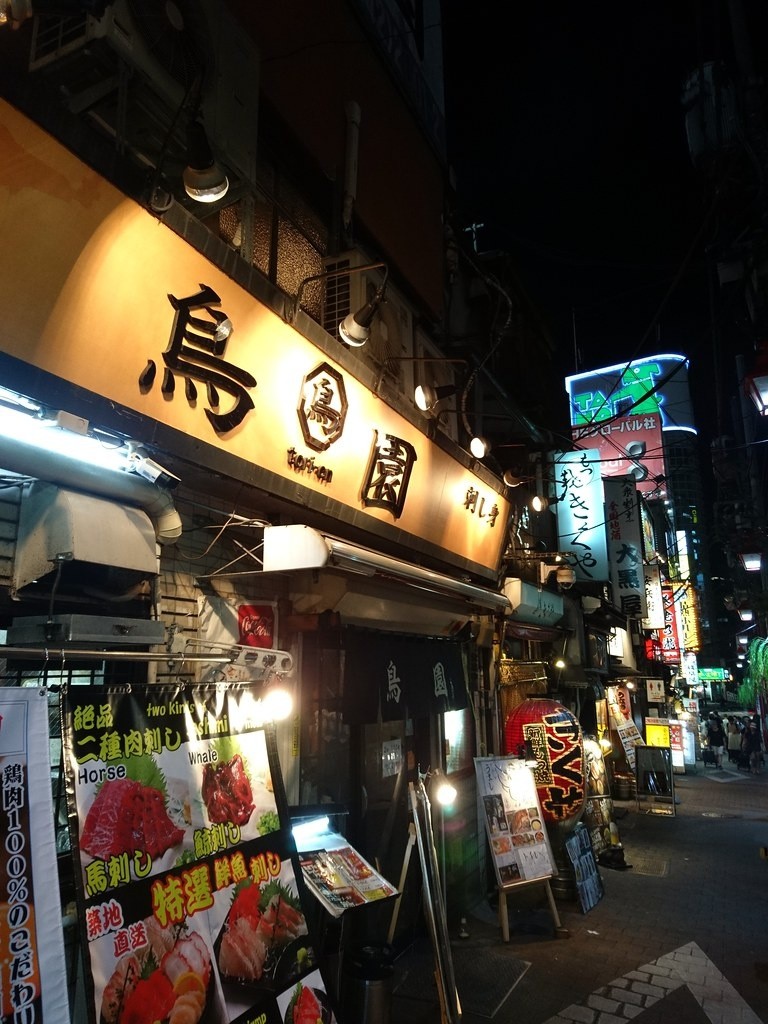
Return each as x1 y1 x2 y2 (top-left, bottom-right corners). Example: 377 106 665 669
271 934 319 986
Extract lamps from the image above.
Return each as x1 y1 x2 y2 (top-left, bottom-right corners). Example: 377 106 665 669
470 435 492 459
430 768 457 806
503 464 522 488
338 292 382 348
414 384 460 411
183 120 231 203
532 495 558 513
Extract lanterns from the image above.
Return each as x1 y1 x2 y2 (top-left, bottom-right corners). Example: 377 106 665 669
506 693 584 825
617 688 630 720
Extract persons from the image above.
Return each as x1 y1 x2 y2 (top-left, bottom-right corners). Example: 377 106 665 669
698 709 765 775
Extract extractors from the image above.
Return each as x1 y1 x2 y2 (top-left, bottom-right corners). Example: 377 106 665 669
7 482 172 649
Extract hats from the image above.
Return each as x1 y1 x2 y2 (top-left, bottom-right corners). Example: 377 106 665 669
750 722 757 729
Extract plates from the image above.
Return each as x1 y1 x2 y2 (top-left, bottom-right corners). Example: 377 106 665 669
100 954 217 1024
283 988 332 1024
210 898 323 1003
